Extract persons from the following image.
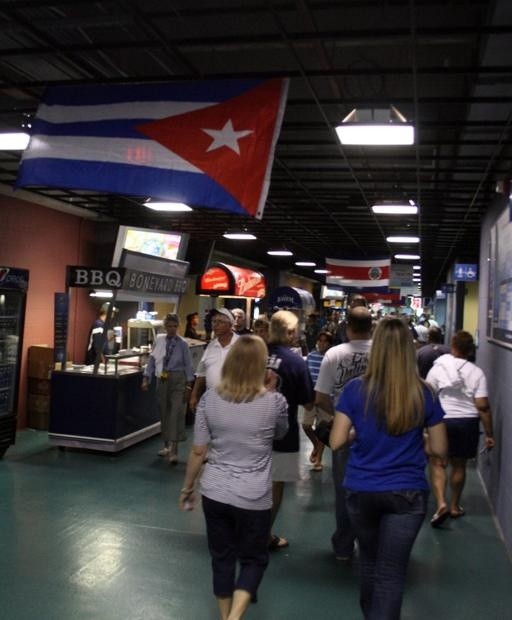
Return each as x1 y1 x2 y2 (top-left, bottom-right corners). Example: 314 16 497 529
141 296 497 620
86 302 119 365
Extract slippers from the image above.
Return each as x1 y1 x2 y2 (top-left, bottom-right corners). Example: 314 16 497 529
451 506 464 518
310 449 322 471
431 511 450 526
268 535 289 547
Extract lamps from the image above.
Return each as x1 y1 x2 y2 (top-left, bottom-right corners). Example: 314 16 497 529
332 105 416 149
223 218 257 242
1 103 32 153
267 238 293 257
386 223 420 244
371 186 419 215
296 257 345 279
394 250 420 282
142 182 194 214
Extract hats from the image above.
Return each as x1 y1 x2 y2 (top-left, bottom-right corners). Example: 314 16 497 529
100 303 119 312
209 307 234 324
419 317 426 324
187 312 198 319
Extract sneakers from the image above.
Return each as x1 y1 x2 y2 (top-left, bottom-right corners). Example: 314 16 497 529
158 447 172 455
170 455 178 462
336 553 350 560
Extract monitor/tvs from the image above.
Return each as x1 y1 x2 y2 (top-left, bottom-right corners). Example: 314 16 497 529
111 225 190 268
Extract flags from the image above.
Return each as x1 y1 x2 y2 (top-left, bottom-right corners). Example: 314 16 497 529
12 76 290 220
325 254 402 308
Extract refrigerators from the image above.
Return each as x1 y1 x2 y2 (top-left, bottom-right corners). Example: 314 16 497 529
0 267 30 458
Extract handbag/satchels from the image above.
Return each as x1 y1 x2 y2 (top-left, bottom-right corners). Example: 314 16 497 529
314 419 333 448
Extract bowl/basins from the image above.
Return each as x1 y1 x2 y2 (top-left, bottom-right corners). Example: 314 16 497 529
71 365 86 370
